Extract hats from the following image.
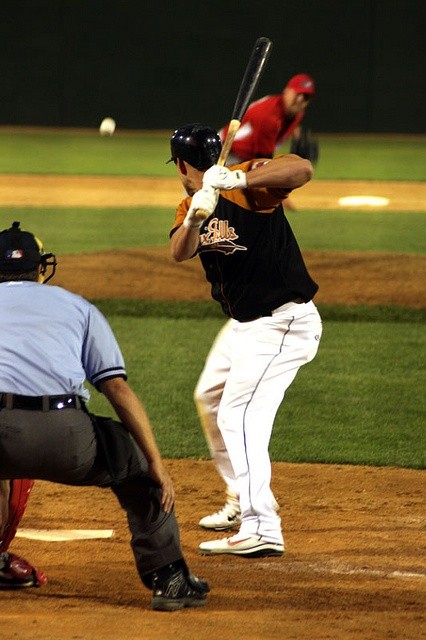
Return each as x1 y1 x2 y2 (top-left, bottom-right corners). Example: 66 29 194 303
286 75 314 93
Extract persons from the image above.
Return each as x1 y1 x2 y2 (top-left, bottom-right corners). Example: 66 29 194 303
169 124 324 557
1 220 210 613
219 75 315 171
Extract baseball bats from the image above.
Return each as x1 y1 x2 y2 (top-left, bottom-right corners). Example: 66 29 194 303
195 37 271 220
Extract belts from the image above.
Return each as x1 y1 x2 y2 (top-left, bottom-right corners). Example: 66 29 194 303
1 392 86 411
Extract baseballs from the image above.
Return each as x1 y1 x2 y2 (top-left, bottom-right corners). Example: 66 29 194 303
98 118 116 136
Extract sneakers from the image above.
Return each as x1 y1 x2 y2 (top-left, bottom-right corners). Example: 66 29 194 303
198 496 279 530
0 553 46 588
198 533 285 556
151 564 208 612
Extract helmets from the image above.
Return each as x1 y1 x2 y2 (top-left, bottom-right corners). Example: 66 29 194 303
165 124 222 171
0 220 56 284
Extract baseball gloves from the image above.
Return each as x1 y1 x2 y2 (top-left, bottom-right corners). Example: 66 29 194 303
290 130 318 164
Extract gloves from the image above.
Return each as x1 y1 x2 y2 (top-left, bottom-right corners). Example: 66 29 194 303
184 189 217 229
203 165 247 191
